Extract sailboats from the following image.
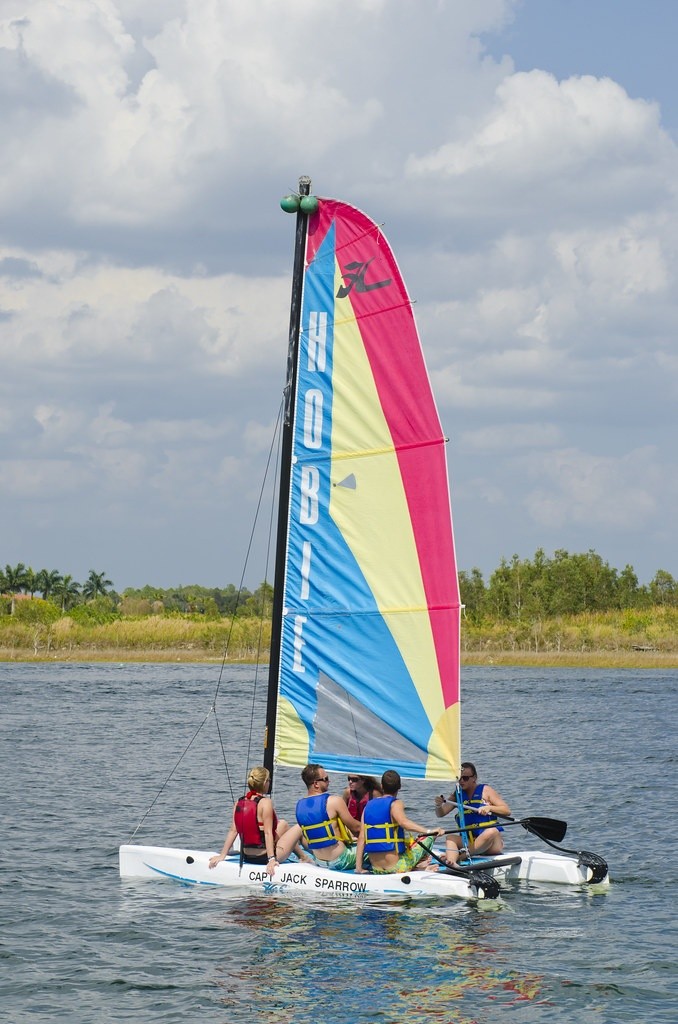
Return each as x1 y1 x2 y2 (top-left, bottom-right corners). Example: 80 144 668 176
117 174 610 901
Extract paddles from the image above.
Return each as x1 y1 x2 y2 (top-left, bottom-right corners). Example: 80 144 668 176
437 794 568 844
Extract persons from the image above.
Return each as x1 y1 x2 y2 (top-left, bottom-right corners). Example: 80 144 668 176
296 764 361 871
352 770 445 874
209 766 317 875
435 762 511 866
342 774 382 836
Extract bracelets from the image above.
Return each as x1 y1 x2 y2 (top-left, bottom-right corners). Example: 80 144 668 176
436 805 442 808
427 829 430 834
268 856 274 861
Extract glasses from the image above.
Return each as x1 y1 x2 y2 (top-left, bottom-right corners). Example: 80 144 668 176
348 776 363 782
267 779 271 783
316 775 328 781
459 775 474 781
